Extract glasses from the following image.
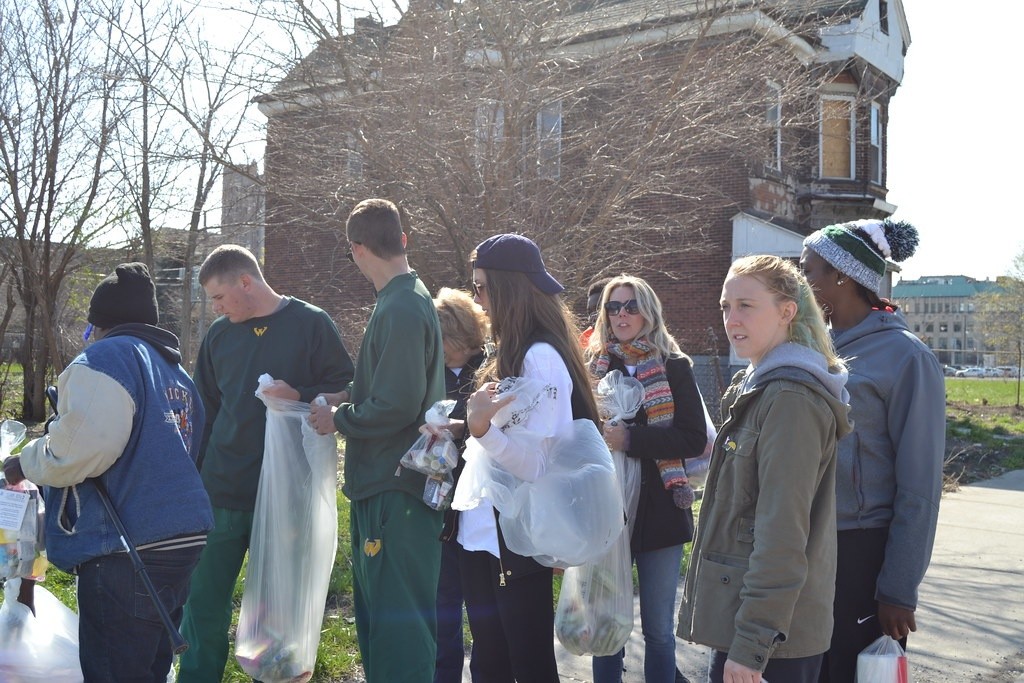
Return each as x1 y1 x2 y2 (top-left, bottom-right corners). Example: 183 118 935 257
604 299 640 315
473 280 488 297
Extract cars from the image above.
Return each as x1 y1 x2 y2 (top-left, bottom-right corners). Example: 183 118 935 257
984 368 1005 377
955 368 987 378
0 316 64 360
944 366 959 377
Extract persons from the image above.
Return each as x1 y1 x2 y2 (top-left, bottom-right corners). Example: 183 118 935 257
582 275 708 683
3 262 214 683
676 251 852 683
303 199 446 683
178 245 352 683
797 219 946 683
419 233 592 683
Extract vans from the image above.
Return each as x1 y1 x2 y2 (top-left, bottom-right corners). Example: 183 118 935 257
998 367 1015 377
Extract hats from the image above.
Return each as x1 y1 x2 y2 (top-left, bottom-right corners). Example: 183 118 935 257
470 232 564 296
87 262 158 328
803 219 920 293
